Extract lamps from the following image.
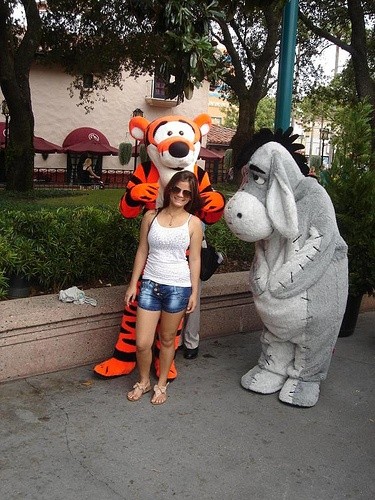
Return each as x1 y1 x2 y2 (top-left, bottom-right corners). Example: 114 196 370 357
320 126 329 166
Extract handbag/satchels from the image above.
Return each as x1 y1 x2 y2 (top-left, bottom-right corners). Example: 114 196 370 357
200 245 220 281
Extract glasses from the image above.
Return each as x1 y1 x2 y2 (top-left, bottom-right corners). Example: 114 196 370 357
171 186 192 197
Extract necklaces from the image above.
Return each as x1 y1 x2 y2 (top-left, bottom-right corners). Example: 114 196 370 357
168 210 185 226
124 171 202 405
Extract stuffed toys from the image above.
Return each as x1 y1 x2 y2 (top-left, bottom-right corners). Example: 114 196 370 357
224 127 349 408
92 113 226 382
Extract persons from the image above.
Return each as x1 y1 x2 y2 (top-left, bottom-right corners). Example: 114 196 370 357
306 166 330 186
79 158 100 190
176 223 206 359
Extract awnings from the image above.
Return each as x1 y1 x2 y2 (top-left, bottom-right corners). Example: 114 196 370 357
0 121 224 160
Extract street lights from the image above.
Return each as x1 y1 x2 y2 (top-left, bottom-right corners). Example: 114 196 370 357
132 108 143 174
319 127 330 166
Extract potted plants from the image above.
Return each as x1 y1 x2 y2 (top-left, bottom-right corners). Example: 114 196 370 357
319 153 375 338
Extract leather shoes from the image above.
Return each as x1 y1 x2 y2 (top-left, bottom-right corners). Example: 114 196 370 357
183 345 199 359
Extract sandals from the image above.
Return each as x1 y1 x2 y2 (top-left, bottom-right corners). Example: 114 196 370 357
127 382 153 401
151 382 169 405
